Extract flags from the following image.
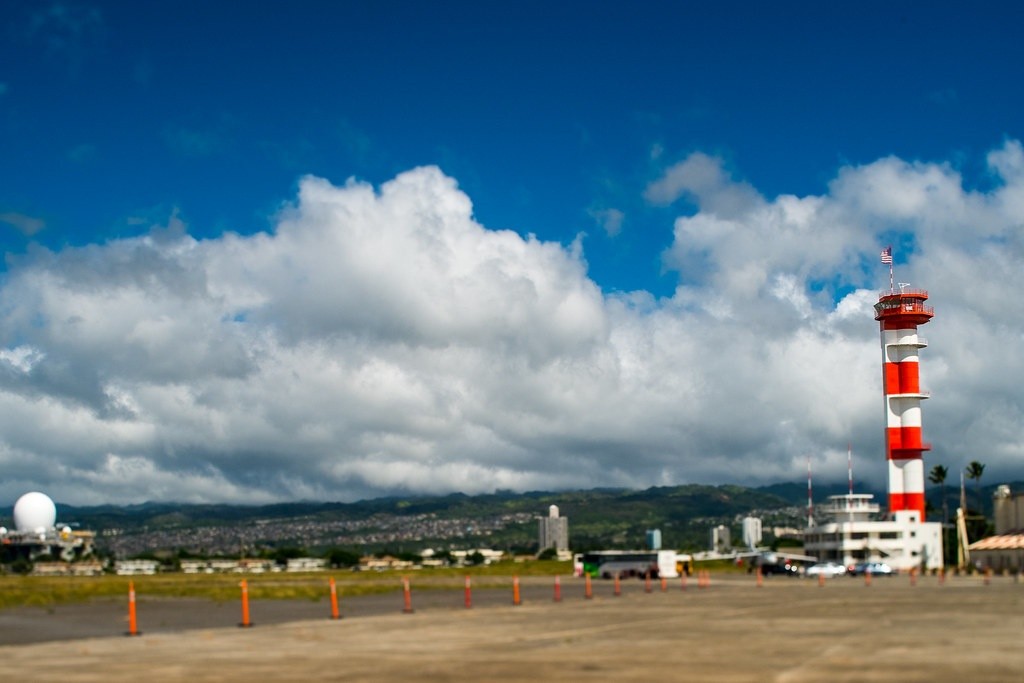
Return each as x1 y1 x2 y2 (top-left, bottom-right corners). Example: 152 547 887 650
881 247 892 265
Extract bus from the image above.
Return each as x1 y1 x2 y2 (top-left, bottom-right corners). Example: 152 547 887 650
676 554 694 577
582 548 679 580
573 553 585 579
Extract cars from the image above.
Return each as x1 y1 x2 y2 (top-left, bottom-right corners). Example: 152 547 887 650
748 559 799 578
804 560 847 579
847 561 896 576
